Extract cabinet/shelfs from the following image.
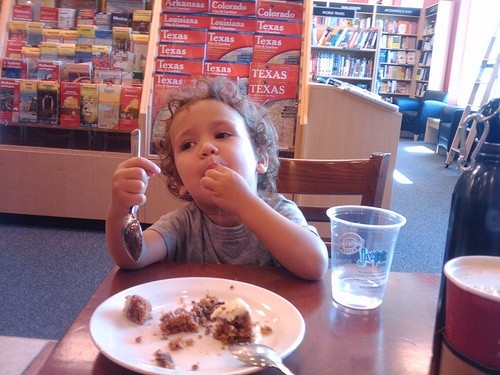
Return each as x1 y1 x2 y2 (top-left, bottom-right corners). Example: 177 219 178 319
1 1 313 234
299 80 404 241
308 0 455 104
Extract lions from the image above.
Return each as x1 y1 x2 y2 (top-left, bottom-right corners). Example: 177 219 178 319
82 92 98 123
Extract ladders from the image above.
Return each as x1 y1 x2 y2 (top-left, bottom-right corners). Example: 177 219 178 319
444 30 500 171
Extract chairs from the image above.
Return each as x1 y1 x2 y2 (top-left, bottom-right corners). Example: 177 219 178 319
272 153 390 265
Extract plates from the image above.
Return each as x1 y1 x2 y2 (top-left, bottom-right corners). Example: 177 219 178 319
89 276 306 375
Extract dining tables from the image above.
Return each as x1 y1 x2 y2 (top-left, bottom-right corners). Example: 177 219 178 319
36 263 443 375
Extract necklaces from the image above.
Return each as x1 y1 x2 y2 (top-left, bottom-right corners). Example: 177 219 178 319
217 207 252 257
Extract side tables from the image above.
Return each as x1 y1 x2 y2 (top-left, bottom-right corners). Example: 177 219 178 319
423 116 441 143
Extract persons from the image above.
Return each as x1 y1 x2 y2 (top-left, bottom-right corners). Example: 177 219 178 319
106 75 329 279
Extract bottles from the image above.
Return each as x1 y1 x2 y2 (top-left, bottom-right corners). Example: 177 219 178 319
427 99 500 375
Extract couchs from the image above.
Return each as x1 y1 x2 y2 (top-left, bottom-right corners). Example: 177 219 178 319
393 90 448 141
436 106 480 155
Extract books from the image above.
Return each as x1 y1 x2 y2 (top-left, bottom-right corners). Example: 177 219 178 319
378 18 418 49
414 16 437 97
311 15 375 49
377 50 415 80
310 53 371 91
376 79 410 104
313 73 399 113
150 0 304 155
0 0 153 131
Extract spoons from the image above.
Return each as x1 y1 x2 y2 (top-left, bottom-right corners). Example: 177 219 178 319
229 344 295 375
121 129 143 262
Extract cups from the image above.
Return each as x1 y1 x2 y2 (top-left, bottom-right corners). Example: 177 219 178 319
326 205 408 312
438 256 500 375
